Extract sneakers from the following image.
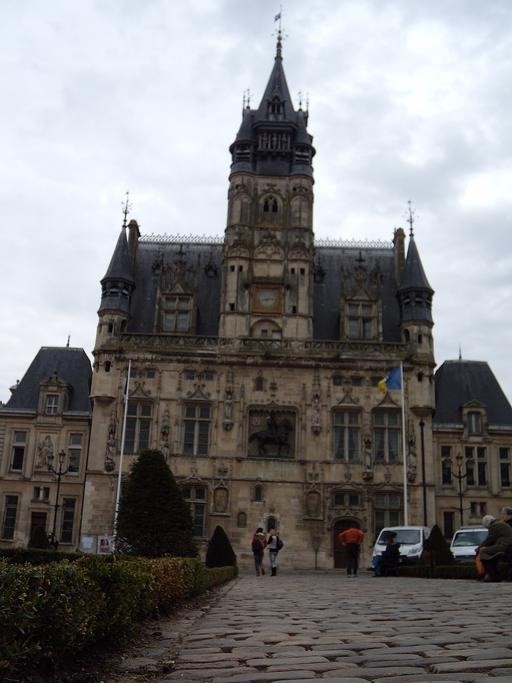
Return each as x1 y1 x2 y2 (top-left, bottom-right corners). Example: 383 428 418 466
261 569 266 575
256 572 260 576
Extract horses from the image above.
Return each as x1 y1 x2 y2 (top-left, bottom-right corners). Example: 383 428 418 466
248 418 292 457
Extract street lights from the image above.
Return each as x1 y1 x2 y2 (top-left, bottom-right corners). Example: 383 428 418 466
439 448 477 525
44 447 78 549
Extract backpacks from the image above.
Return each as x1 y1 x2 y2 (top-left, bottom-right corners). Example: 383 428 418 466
276 536 284 551
254 538 263 553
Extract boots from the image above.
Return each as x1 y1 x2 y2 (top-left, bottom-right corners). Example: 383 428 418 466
274 567 277 576
271 567 274 576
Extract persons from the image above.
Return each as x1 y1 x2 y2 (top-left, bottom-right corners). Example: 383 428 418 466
499 506 511 524
338 523 365 578
252 527 268 576
266 528 278 575
474 513 511 582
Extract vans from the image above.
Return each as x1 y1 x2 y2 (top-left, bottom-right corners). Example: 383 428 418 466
448 524 491 563
368 523 434 571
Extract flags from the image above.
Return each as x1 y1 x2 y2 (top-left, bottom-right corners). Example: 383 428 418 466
376 367 404 393
123 365 132 398
